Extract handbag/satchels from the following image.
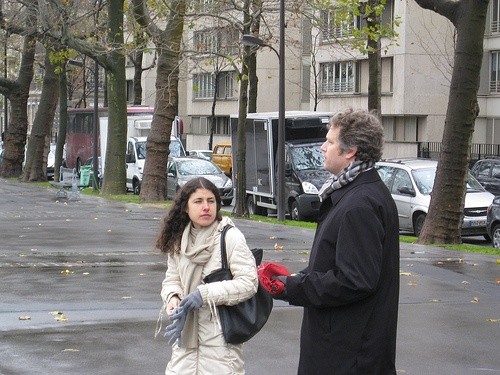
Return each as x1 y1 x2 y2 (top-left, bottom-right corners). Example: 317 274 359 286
204 225 273 345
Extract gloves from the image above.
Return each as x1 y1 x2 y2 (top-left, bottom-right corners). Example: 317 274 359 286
178 290 203 312
270 274 288 302
165 307 187 348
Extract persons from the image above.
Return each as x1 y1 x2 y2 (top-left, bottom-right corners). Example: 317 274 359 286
156 178 258 375
270 110 400 375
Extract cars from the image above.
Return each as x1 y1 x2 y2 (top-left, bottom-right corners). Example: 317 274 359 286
166 156 234 207
486 196 500 249
374 158 495 242
189 150 213 161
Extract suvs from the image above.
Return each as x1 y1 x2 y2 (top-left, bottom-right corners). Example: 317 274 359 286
471 156 500 198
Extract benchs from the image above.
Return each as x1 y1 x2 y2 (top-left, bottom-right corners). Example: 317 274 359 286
49 166 81 202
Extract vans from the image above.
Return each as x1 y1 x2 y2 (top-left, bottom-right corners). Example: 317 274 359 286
212 144 232 178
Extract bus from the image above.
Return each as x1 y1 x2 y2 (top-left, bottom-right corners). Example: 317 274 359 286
66 106 183 179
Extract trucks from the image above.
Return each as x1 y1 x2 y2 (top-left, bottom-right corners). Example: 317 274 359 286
99 117 189 195
230 111 335 221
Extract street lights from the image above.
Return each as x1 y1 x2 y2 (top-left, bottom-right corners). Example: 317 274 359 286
240 34 285 222
67 59 99 191
